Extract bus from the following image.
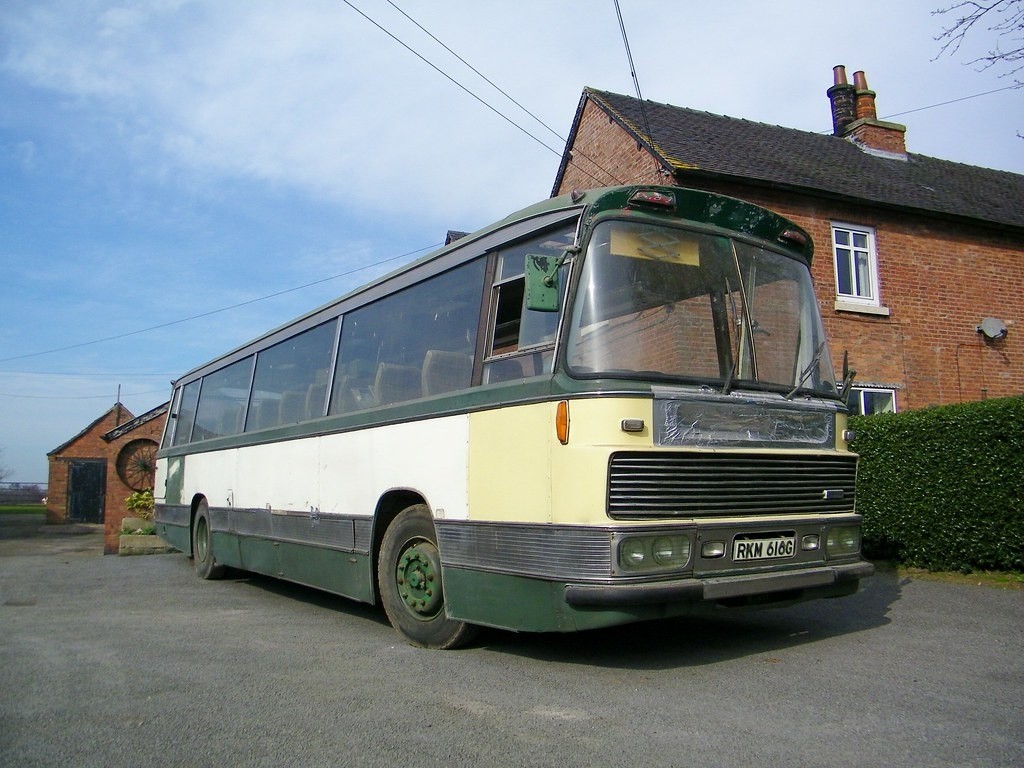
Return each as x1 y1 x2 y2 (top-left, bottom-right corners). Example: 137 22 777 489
151 185 876 652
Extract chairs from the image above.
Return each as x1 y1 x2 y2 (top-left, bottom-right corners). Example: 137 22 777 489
421 349 473 397
338 375 376 414
278 390 306 426
305 385 338 419
176 406 258 445
256 398 280 430
489 360 523 384
374 362 421 407
